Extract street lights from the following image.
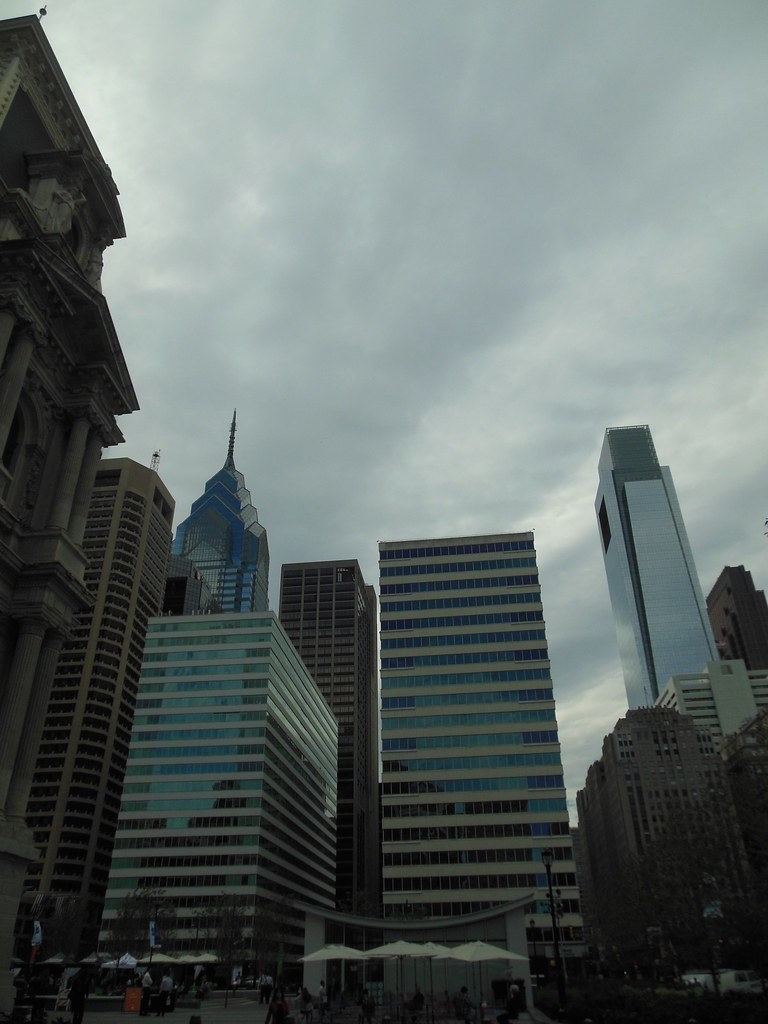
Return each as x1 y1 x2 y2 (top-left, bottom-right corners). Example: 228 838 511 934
541 847 575 1017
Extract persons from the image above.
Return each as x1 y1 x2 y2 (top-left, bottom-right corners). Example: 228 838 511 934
357 989 375 1023
401 986 424 1023
497 985 521 1023
294 987 317 1024
265 989 289 1024
155 970 173 1017
319 980 327 1015
259 971 273 1003
453 986 477 1024
66 959 90 1024
330 976 342 1013
139 966 153 1016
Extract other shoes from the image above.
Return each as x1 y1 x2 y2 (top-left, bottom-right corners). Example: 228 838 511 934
140 1013 152 1016
156 1014 159 1017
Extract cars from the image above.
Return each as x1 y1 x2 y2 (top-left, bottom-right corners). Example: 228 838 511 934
230 973 259 990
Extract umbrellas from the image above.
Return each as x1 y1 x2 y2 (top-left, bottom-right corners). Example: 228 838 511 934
10 949 225 974
298 941 530 1024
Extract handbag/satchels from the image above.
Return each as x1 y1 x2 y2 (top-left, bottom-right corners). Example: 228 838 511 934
322 996 328 1003
286 1016 294 1024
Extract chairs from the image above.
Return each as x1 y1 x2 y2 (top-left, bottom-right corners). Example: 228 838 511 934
300 999 519 1024
